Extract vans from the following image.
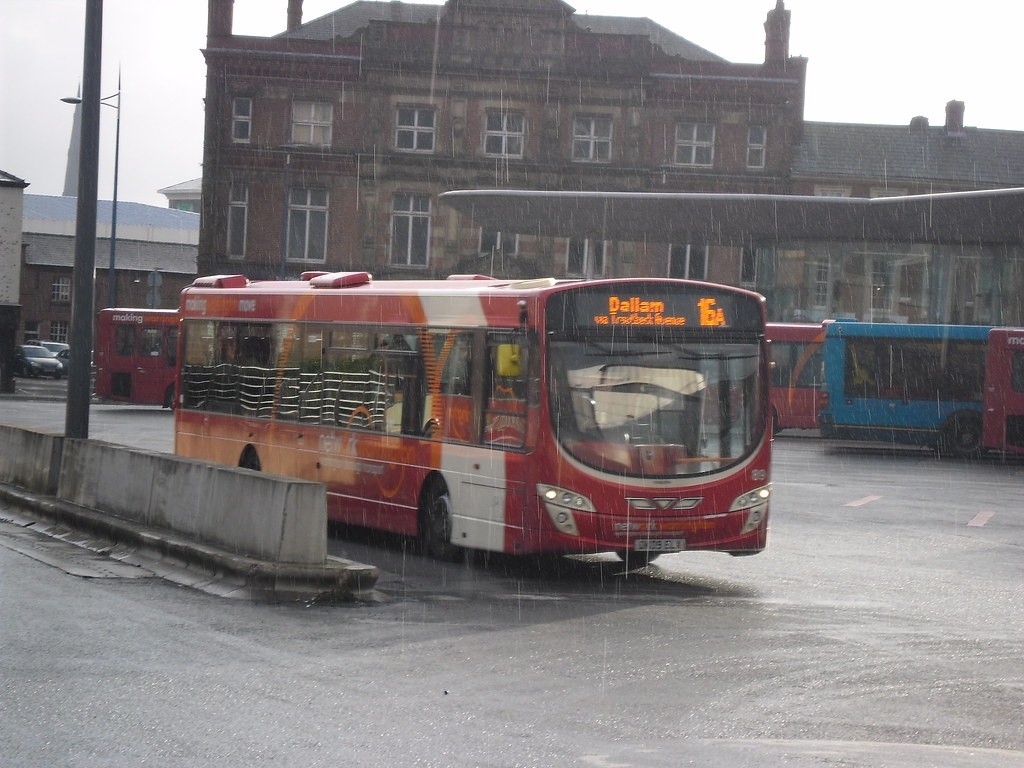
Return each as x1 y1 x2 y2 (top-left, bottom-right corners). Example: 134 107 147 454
23 337 70 359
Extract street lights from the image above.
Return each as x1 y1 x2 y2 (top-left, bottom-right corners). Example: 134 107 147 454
59 84 123 309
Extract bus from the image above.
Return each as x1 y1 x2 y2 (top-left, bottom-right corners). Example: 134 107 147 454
704 317 837 436
170 266 783 568
982 329 1024 456
815 317 1023 461
94 307 179 409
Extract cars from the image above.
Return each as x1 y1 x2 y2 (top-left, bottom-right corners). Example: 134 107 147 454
12 345 64 380
55 349 70 374
